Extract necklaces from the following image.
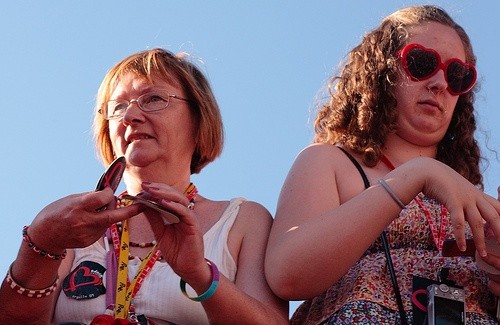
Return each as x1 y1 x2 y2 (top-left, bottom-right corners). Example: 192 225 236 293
117 192 196 262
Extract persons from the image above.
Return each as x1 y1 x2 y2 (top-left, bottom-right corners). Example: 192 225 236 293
0 47 290 325
263 5 500 325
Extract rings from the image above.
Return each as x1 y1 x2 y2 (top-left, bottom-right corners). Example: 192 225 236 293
187 202 195 211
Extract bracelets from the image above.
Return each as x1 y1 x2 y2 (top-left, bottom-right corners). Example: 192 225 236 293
179 258 219 302
6 262 59 298
378 178 408 209
21 224 68 260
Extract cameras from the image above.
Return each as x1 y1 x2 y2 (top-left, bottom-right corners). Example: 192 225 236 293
428 284 466 325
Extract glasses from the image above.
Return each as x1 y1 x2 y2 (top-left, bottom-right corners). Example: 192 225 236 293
97 90 195 121
396 43 478 96
